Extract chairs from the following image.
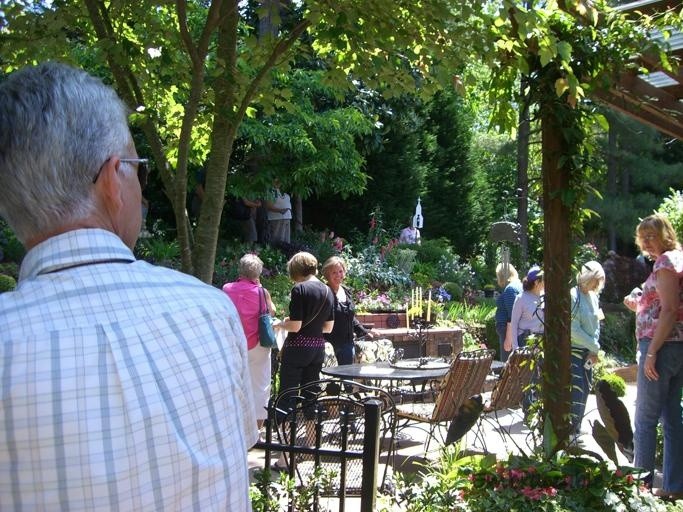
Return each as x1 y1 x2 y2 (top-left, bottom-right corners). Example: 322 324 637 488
347 340 419 442
419 322 474 405
313 338 358 452
459 344 547 455
262 373 402 511
377 350 496 493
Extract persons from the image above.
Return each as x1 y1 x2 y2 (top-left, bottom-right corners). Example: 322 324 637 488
622 213 683 502
494 261 522 410
569 261 606 446
221 253 276 443
0 62 258 512
138 165 421 245
510 265 544 428
321 256 378 434
272 250 336 474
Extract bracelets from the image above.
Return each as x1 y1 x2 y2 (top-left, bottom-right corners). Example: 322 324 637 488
645 352 657 359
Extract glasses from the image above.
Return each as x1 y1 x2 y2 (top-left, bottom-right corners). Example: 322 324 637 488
92 158 149 191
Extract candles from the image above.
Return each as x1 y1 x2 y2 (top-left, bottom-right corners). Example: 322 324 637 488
420 286 423 314
427 290 431 322
415 285 419 314
405 301 409 332
412 288 415 320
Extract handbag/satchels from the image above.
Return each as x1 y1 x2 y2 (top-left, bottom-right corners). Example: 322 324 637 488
258 287 276 347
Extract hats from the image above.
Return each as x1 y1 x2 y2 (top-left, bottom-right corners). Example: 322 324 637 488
528 269 544 281
